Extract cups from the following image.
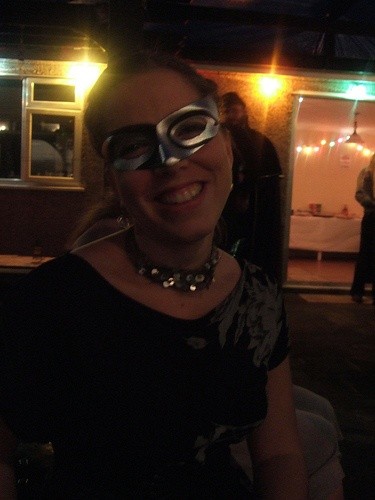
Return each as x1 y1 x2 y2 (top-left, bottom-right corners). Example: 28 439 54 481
310 204 321 214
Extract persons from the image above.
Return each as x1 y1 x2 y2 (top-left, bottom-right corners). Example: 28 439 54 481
0 42 309 500
216 92 375 304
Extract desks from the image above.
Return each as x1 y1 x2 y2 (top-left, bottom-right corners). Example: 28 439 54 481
291 216 362 261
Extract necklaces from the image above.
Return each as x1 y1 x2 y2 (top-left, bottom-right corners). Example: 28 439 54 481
126 222 220 294
